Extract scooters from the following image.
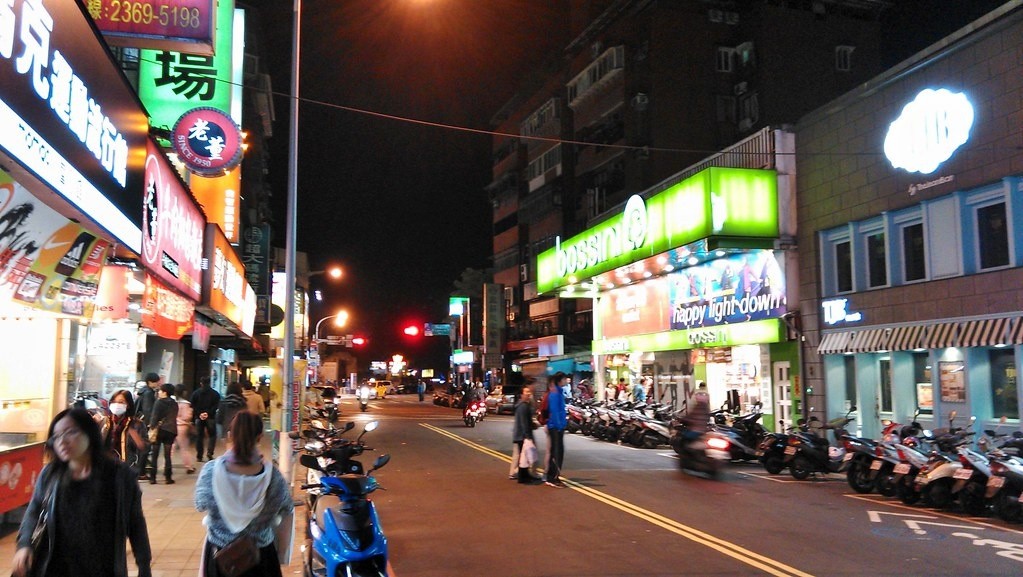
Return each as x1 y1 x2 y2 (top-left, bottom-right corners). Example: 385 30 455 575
562 396 1011 527
477 400 488 422
286 387 391 577
460 390 478 428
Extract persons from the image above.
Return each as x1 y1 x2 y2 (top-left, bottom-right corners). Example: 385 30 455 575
509 371 654 490
129 372 270 484
446 378 487 412
92 390 151 477
195 411 291 577
418 381 423 401
10 408 152 577
421 379 426 399
656 351 693 416
694 383 710 411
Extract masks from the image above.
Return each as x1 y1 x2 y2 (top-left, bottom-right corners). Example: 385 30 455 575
108 403 127 415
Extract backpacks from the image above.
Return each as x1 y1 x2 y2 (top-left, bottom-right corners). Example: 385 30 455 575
536 389 558 425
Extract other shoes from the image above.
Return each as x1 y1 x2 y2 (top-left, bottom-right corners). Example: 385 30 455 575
197 458 204 462
206 452 214 460
165 476 175 484
519 471 542 485
186 468 196 474
150 474 157 484
544 478 564 488
139 474 150 482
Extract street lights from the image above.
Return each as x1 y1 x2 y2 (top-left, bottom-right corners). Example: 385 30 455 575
315 310 348 352
301 267 342 360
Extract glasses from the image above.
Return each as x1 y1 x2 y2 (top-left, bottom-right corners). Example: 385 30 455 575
47 427 79 446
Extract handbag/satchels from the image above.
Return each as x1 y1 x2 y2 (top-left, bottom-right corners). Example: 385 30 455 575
214 536 261 575
147 428 158 443
520 439 539 468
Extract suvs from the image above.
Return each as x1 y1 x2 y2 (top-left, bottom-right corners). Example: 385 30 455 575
484 385 516 415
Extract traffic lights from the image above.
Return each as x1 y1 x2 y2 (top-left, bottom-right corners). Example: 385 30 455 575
403 325 419 336
352 338 364 345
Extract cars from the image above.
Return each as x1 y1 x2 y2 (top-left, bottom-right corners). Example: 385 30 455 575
381 381 395 395
355 378 388 401
